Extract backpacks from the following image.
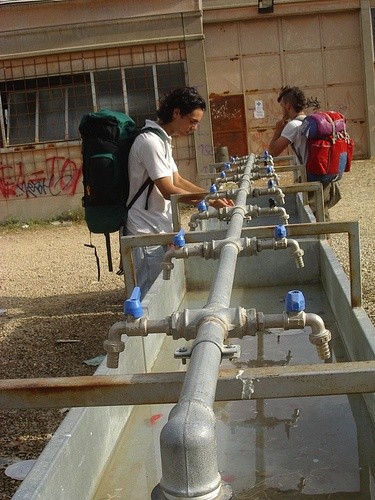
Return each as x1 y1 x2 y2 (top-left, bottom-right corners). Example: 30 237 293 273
78 109 137 234
300 110 353 183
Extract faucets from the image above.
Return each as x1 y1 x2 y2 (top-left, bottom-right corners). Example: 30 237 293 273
215 171 234 187
227 157 241 166
256 224 306 270
222 163 237 175
259 166 280 186
258 197 290 227
257 290 332 361
188 199 219 231
104 285 171 369
259 179 287 205
231 154 243 162
260 150 273 160
259 156 275 168
204 183 227 206
260 160 275 177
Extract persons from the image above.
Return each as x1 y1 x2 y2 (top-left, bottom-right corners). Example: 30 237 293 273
128 85 235 303
269 85 335 223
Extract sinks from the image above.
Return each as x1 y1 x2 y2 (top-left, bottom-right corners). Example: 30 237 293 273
182 186 321 241
6 238 375 500
163 228 203 281
